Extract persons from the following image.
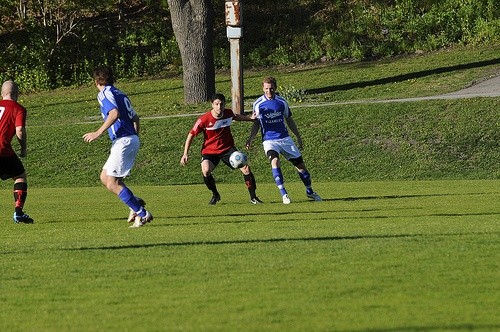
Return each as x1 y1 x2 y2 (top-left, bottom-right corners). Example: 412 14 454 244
245 76 322 204
0 81 34 224
81 65 154 228
180 94 265 206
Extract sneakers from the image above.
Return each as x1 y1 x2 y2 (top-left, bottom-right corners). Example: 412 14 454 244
306 192 322 201
127 197 146 222
130 211 153 228
250 197 263 204
13 212 34 224
208 194 220 205
283 194 291 204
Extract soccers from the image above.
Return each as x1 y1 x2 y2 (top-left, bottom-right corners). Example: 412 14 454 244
229 151 247 169
